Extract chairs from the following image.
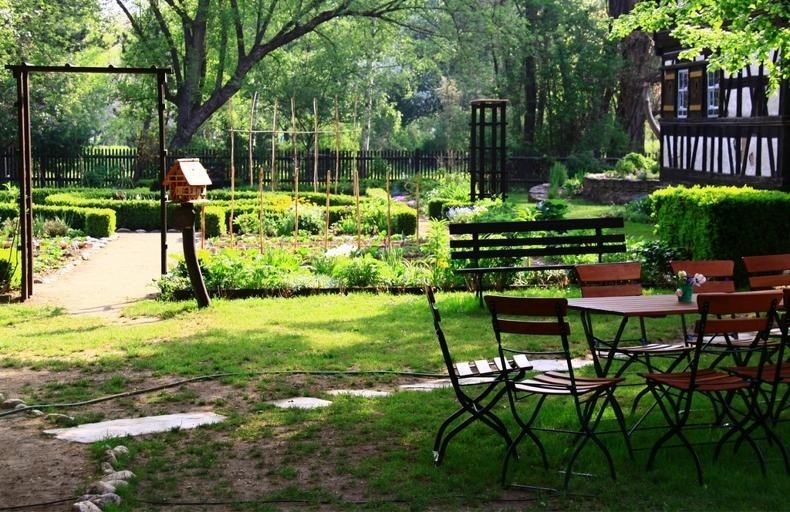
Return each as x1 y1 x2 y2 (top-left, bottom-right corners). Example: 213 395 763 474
426 283 533 466
635 291 784 484
484 295 626 494
565 255 790 463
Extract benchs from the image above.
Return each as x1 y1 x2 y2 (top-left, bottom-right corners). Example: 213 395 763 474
449 217 629 309
